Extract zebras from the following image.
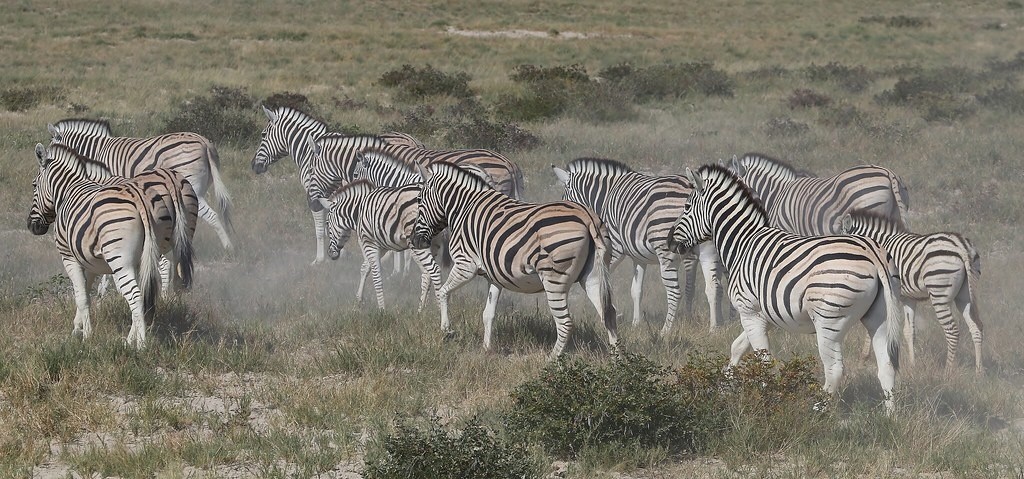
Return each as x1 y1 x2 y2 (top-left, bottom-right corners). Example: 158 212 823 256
252 104 987 414
27 118 235 349
